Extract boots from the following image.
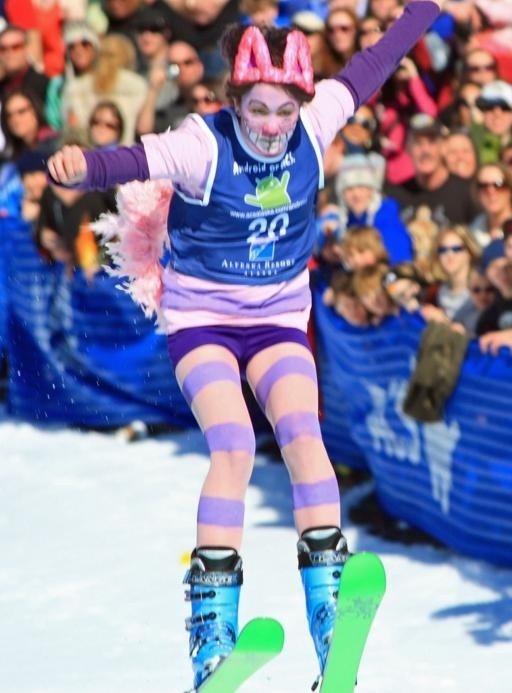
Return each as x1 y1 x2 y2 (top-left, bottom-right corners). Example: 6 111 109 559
295 527 354 692
184 546 243 693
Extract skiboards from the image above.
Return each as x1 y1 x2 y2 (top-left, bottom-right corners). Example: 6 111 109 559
193 552 386 692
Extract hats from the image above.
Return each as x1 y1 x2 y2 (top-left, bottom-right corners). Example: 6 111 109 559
223 23 315 101
334 152 383 194
408 114 439 134
476 79 512 109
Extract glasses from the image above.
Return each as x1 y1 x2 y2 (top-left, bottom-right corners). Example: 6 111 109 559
468 63 496 72
349 116 372 128
472 286 493 292
0 26 200 130
325 25 351 33
436 244 464 254
477 180 509 192
